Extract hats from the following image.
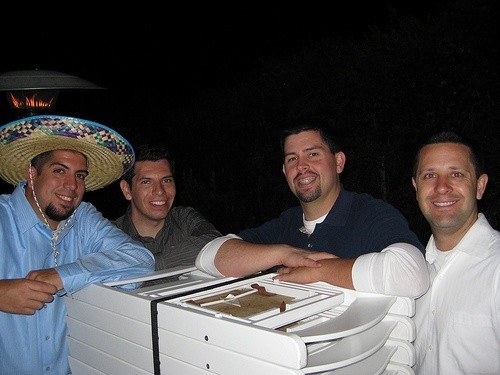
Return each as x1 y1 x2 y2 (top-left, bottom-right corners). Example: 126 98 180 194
0 116 135 192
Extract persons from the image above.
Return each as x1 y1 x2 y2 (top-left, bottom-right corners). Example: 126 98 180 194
0 115 156 374
410 132 500 375
195 120 430 300
110 146 223 274
